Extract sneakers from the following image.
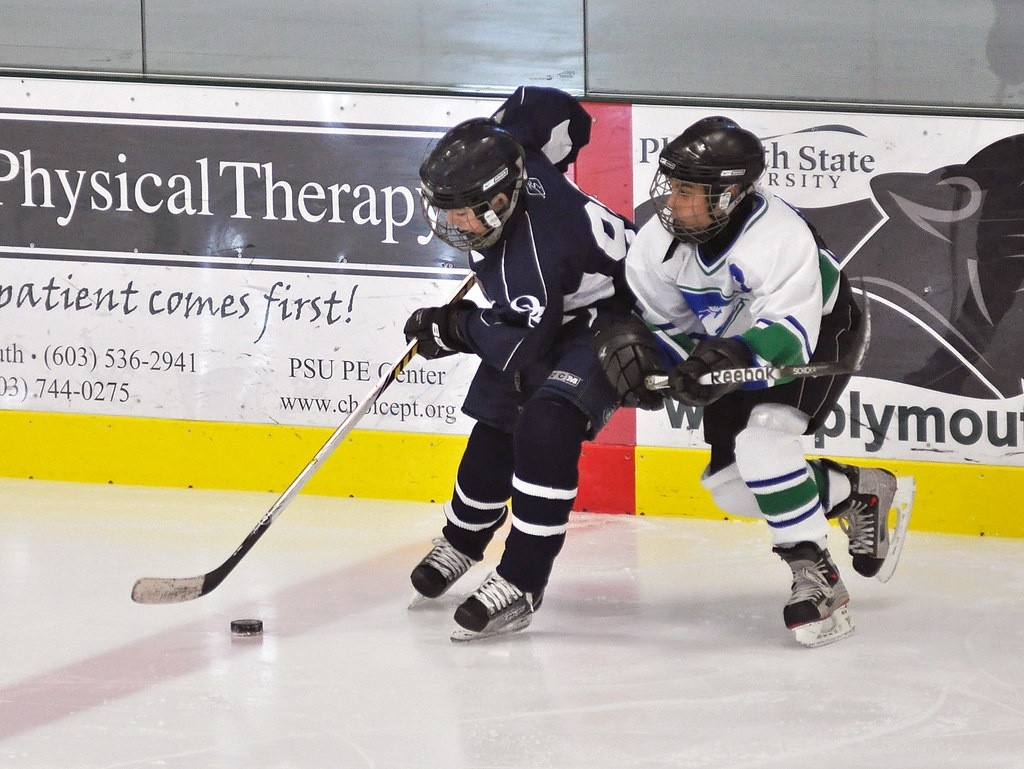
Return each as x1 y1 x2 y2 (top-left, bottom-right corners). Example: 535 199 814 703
770 540 855 646
450 571 546 640
407 537 484 607
819 457 913 584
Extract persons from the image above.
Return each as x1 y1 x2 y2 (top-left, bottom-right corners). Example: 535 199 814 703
403 84 638 644
626 116 916 647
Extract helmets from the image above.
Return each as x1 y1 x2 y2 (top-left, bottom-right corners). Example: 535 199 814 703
418 117 526 251
649 116 764 242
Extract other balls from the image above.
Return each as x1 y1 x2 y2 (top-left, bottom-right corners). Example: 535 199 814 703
230 619 263 637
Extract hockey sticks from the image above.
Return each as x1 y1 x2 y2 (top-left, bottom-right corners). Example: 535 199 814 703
642 276 873 392
128 270 475 606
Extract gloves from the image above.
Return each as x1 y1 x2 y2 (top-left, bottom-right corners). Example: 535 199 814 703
403 299 475 359
595 321 667 411
668 337 753 407
